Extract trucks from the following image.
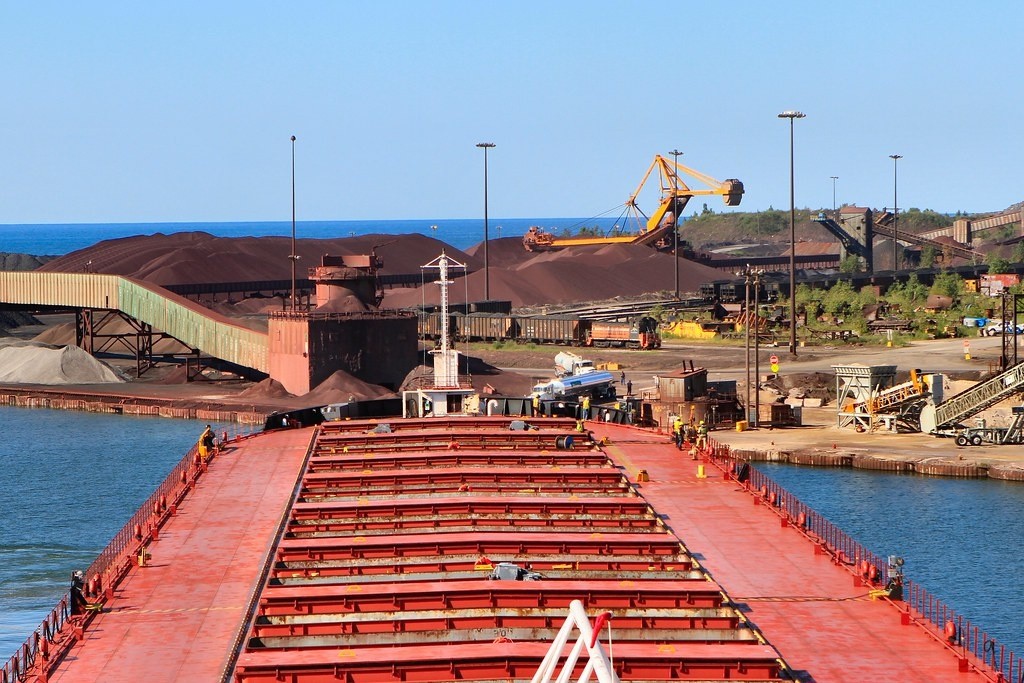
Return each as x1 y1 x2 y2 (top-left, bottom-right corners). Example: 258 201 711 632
554 350 596 379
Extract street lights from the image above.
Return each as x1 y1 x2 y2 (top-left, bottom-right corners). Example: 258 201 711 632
668 148 684 295
889 154 904 270
736 263 766 428
290 135 296 311
830 176 839 217
778 110 807 349
475 142 496 302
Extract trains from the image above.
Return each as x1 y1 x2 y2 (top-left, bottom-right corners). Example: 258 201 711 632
410 310 662 352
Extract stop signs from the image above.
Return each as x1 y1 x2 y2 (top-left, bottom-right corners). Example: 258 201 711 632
770 357 778 365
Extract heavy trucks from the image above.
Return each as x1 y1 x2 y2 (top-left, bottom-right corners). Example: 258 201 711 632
527 370 617 408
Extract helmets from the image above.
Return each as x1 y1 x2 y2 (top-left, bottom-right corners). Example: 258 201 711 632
677 417 680 420
700 421 704 424
206 425 211 428
585 397 589 400
679 422 684 425
536 394 541 398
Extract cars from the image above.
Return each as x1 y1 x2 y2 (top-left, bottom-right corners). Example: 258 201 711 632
988 321 1023 337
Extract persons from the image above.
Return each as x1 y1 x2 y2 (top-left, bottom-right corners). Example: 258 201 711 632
614 401 622 411
626 380 633 396
620 371 626 385
670 411 707 461
532 394 541 418
583 397 591 421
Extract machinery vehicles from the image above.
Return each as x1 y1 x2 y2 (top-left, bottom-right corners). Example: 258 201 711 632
521 152 745 260
